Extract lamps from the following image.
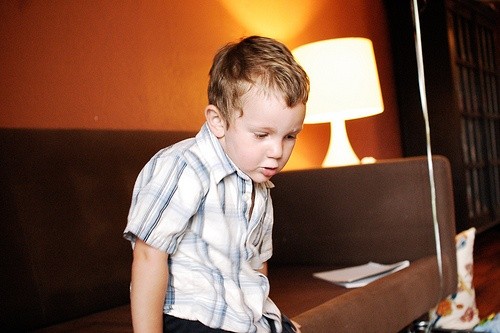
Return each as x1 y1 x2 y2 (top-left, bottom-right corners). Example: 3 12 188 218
291 37 384 168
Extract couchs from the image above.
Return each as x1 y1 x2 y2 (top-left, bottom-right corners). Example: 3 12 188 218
0 127 456 333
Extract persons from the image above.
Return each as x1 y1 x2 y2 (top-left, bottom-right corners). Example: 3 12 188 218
122 35 309 333
